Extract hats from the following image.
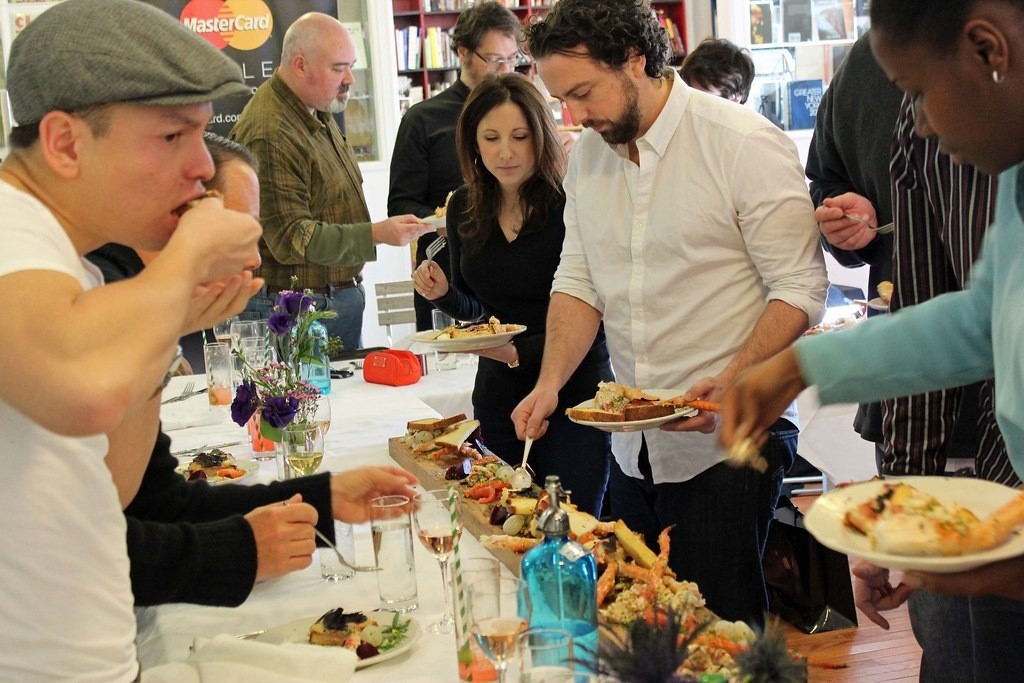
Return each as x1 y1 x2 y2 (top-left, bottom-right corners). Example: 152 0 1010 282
5 1 254 124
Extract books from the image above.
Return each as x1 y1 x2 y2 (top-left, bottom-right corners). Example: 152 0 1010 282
391 0 684 126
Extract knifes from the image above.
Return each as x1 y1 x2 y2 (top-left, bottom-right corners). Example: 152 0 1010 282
180 388 207 401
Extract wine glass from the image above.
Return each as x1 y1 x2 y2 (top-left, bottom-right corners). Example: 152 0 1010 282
293 394 331 451
412 489 465 634
465 576 531 683
212 315 241 399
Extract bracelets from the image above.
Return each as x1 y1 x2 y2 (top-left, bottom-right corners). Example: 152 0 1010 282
508 359 519 369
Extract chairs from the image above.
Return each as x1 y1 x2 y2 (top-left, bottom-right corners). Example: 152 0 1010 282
375 281 417 351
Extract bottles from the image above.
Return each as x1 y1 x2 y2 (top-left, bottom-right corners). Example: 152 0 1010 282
291 321 332 396
516 475 599 683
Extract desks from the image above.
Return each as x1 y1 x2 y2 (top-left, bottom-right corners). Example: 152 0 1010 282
795 305 880 495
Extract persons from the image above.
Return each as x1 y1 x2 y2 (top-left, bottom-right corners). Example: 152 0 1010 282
407 71 615 523
224 11 438 354
802 25 1024 683
518 0 830 641
81 132 421 606
0 0 265 683
678 37 755 104
387 1 523 331
717 0 1024 683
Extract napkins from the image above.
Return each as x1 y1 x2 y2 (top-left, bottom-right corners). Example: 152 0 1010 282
187 634 357 683
161 408 229 430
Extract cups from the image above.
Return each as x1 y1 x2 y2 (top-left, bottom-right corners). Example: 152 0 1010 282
203 342 233 407
317 471 357 583
516 628 575 683
249 411 277 461
449 556 501 683
280 423 325 476
367 494 420 615
230 319 268 402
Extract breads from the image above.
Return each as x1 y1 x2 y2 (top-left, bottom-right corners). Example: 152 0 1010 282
567 399 676 422
309 609 377 647
188 453 235 477
506 497 675 576
450 316 520 339
408 414 480 450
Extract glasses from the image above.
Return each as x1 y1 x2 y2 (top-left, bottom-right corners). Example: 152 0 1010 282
470 50 525 69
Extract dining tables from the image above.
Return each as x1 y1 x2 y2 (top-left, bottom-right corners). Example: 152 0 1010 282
133 352 531 683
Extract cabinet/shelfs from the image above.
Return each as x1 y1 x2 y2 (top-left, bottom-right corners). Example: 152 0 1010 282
393 0 688 124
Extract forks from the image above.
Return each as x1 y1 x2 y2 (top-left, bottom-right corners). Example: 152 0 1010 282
349 359 363 369
426 235 446 267
162 381 195 405
283 502 383 573
842 212 895 234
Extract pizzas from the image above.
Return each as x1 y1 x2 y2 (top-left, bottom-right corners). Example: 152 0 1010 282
843 484 976 532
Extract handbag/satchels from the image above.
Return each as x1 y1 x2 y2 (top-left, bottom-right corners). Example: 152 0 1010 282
759 451 859 634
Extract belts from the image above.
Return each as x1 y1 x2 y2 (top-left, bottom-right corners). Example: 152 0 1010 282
295 272 363 296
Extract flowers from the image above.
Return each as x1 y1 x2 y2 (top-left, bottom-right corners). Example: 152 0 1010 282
230 274 343 443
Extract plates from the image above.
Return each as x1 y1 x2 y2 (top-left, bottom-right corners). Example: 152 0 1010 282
409 323 527 353
253 609 422 671
867 298 888 312
568 388 697 433
803 474 1024 573
421 215 446 229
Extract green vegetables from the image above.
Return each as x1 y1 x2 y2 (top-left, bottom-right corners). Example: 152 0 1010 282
377 612 411 653
